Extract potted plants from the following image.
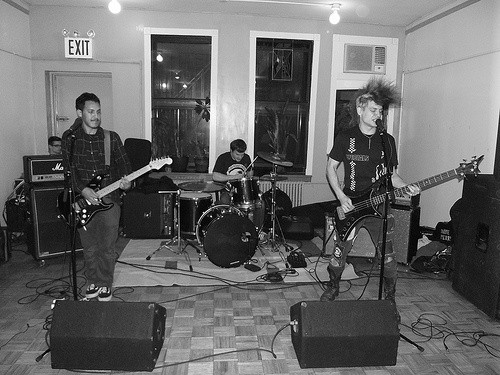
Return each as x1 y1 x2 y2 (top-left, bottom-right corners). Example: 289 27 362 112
168 130 189 173
194 97 208 173
263 100 298 173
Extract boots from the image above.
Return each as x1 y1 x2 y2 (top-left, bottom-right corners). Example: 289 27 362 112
383 276 401 323
320 265 344 301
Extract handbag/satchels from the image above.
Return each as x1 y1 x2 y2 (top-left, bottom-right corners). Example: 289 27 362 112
425 247 450 274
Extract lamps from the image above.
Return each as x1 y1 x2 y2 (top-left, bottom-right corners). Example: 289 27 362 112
61 27 96 60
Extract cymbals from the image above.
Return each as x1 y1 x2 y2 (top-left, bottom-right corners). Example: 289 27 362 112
259 176 288 181
256 151 293 167
177 181 227 192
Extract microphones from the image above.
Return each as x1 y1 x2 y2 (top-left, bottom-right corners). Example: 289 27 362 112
65 119 83 137
376 119 386 135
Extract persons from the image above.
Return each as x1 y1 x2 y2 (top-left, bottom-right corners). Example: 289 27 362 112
213 139 254 212
48 136 61 155
320 75 421 300
61 92 134 302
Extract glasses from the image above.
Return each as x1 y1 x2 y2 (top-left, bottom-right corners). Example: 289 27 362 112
51 144 61 148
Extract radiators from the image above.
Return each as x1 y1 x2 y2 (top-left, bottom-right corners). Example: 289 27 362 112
259 180 304 209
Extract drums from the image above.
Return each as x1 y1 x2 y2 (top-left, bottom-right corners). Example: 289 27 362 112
229 177 262 204
196 204 259 266
176 192 211 237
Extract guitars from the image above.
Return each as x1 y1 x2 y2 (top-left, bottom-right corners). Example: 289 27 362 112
57 156 173 230
334 153 484 241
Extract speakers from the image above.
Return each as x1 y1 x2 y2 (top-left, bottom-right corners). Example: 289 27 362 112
448 173 500 323
121 190 174 240
324 212 376 258
390 205 422 265
290 300 400 368
23 184 86 261
50 300 167 372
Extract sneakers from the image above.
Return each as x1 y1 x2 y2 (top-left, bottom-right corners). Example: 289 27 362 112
85 282 112 302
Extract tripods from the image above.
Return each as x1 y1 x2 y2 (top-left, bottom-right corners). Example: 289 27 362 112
145 195 201 272
258 163 294 268
